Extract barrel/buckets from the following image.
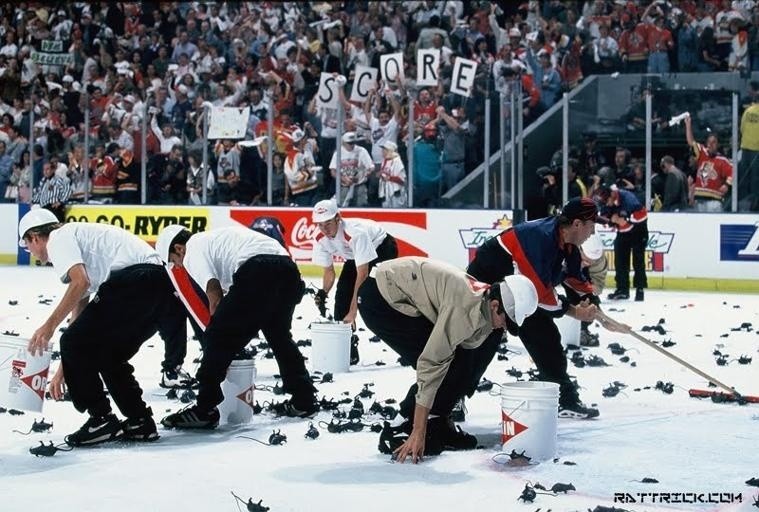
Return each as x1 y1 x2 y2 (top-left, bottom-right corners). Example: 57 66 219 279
310 322 352 373
215 359 256 425
500 381 561 462
552 315 583 350
0 334 55 413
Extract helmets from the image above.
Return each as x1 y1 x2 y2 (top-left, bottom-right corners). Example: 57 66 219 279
154 225 185 269
312 199 341 224
19 209 59 240
504 275 539 327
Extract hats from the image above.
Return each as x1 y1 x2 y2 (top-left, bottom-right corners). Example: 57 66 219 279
380 140 396 152
58 10 65 17
62 75 73 83
178 84 188 94
34 8 48 22
344 132 357 142
509 28 522 39
563 198 610 224
124 95 135 104
292 129 306 143
82 13 92 20
592 187 611 207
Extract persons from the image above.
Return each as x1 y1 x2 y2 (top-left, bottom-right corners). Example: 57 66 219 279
2 2 757 211
1 199 652 465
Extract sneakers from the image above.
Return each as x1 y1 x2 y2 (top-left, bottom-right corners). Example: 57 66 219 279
163 366 200 388
428 418 478 450
559 402 600 418
608 287 629 299
69 415 124 445
636 289 643 302
581 330 599 346
349 335 359 365
162 406 220 429
378 421 444 455
274 400 318 418
119 416 160 441
